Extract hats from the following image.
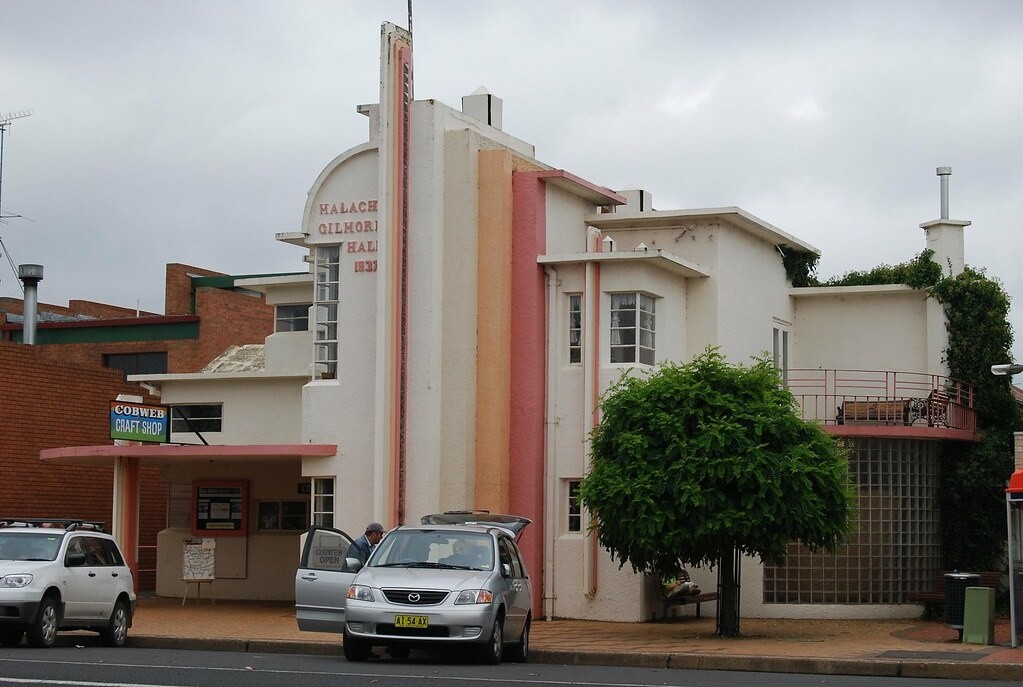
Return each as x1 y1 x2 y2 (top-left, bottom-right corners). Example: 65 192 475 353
366 522 388 533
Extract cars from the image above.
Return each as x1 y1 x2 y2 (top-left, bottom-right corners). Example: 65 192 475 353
294 508 533 665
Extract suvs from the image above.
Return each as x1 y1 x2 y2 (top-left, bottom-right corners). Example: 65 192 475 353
0 517 137 649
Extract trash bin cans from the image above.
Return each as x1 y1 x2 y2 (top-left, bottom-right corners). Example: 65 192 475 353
942 570 981 643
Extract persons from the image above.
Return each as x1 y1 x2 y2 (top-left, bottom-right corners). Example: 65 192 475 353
344 522 388 659
661 564 701 599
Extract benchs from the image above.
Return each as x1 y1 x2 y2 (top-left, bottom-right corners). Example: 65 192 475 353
907 389 952 426
835 401 908 426
655 568 717 619
907 570 1006 620
